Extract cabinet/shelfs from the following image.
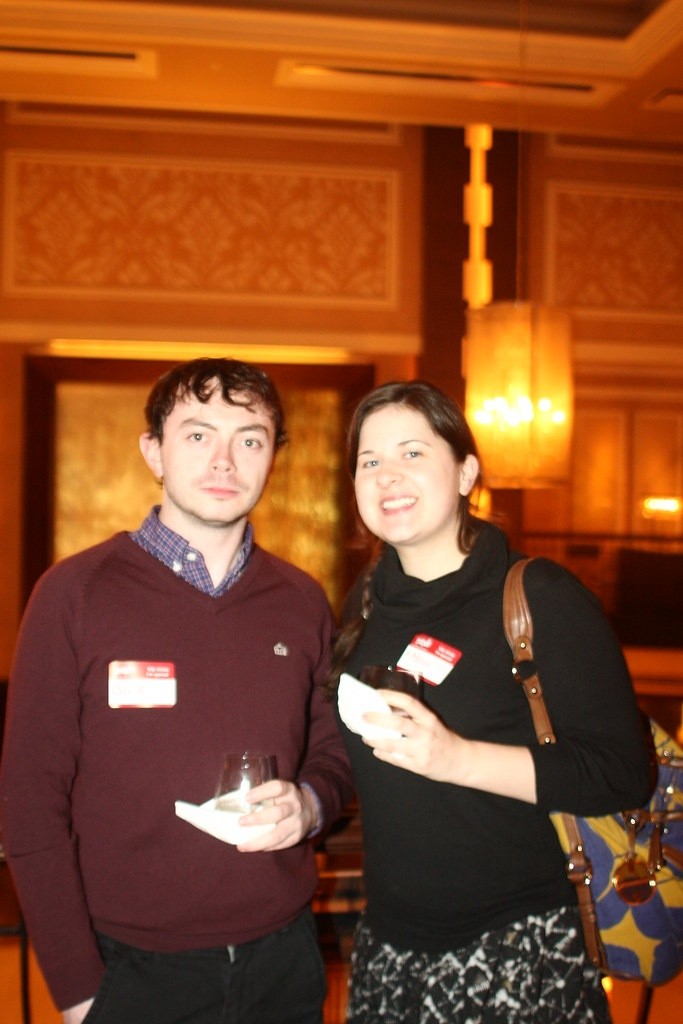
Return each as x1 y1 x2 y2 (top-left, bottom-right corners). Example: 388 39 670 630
522 400 682 613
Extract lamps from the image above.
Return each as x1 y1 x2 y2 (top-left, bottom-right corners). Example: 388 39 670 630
464 296 576 491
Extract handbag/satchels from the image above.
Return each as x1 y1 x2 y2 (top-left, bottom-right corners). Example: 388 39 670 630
503 557 683 985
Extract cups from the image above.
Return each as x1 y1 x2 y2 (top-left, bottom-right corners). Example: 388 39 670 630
217 753 275 821
375 667 420 725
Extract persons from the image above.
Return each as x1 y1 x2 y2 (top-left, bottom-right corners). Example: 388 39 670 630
0 359 354 1024
334 381 661 1024
